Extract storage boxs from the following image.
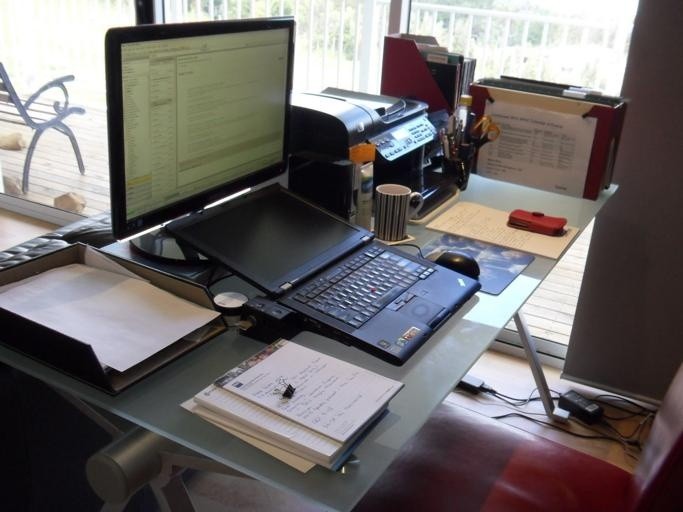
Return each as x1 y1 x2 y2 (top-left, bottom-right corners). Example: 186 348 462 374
462 76 630 201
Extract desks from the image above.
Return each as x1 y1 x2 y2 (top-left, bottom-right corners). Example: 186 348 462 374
0 168 619 511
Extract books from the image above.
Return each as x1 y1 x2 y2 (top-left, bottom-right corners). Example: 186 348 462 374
417 41 476 110
193 336 405 470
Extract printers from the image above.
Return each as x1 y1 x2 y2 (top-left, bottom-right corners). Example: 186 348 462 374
288 85 446 222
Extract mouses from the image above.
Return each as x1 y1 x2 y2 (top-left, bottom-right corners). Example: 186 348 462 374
435 250 480 282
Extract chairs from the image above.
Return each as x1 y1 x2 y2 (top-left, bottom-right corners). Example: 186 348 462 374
353 358 683 512
0 63 85 193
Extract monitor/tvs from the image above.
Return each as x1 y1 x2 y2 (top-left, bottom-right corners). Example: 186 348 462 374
104 13 296 273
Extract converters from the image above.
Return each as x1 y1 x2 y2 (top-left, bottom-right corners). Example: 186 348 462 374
558 389 606 425
457 373 487 395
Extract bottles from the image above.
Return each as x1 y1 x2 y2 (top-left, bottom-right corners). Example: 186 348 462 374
448 94 476 136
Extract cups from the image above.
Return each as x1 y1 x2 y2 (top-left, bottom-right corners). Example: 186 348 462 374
375 184 426 241
441 154 473 191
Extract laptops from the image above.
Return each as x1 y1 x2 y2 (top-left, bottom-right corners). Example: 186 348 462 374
164 180 484 368
